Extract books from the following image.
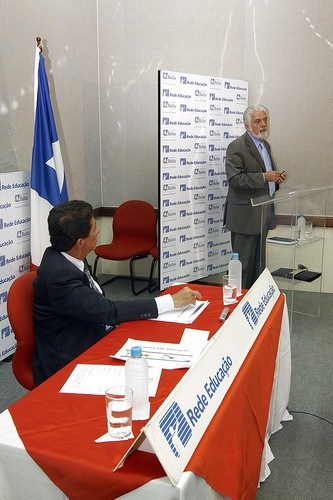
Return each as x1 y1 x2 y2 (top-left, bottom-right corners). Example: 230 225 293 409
148 300 210 324
59 364 162 397
271 267 322 283
109 339 203 370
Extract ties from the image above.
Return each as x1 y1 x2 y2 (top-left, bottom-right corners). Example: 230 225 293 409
259 143 275 198
82 262 100 294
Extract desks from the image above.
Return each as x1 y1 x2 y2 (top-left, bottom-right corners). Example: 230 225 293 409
0 281 292 500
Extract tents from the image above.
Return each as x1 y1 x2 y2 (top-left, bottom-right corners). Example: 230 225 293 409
267 236 298 245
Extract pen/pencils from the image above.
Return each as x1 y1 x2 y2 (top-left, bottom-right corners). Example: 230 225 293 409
280 170 285 176
190 303 205 316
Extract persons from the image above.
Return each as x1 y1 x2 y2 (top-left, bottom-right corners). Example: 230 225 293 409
31 199 203 387
223 105 287 290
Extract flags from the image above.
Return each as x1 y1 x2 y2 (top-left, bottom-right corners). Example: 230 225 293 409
30 48 68 272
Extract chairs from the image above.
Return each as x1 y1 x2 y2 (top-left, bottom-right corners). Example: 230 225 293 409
147 245 160 295
89 199 157 297
6 271 37 391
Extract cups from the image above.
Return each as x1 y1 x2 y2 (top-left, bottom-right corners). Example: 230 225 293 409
305 222 312 237
291 226 301 240
222 275 237 303
105 386 134 440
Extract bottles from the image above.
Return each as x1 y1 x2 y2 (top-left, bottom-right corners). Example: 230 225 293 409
125 346 149 412
228 253 241 297
298 213 306 240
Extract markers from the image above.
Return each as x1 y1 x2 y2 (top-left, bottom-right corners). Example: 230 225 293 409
219 307 229 322
